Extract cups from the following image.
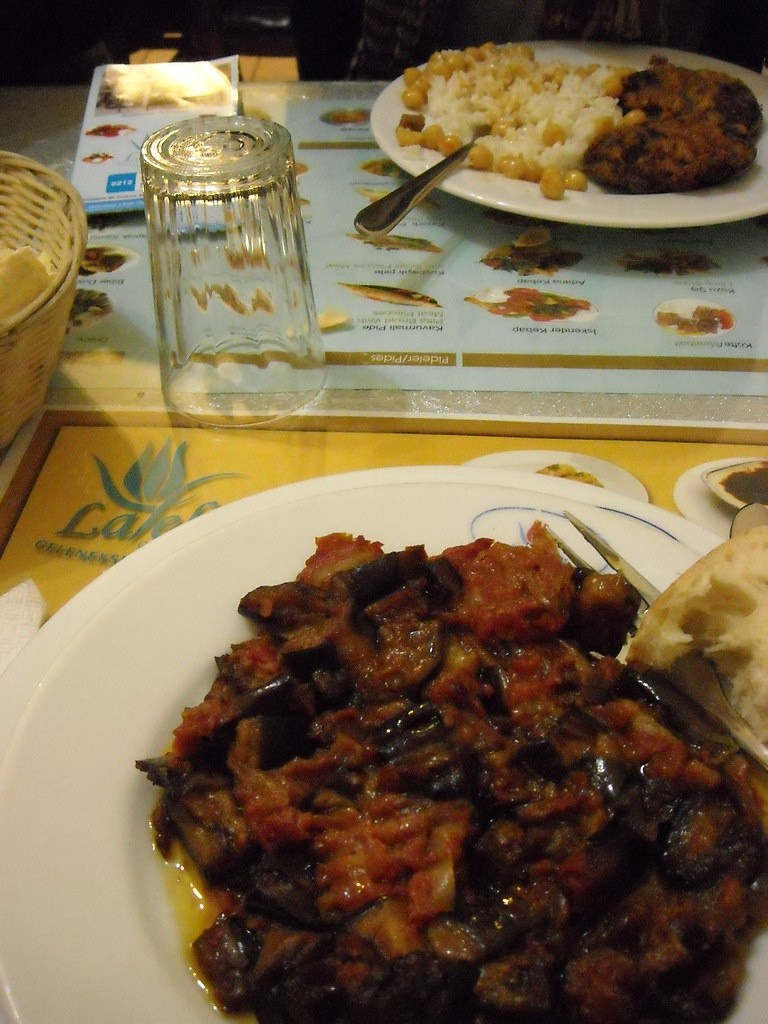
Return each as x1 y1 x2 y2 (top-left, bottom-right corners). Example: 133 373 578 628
138 117 329 429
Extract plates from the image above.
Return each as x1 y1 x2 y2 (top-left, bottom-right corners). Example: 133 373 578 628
462 450 649 504
700 459 768 510
654 298 735 339
2 466 768 1024
475 289 600 324
673 457 768 539
370 39 768 229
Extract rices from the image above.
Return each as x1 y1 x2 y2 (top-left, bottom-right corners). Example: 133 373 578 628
420 46 631 176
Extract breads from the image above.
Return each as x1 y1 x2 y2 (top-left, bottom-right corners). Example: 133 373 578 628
0 245 51 322
623 523 768 770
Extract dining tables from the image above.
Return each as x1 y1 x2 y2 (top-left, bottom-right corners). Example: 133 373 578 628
0 81 768 1024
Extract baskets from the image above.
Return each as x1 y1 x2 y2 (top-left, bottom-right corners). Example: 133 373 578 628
0 150 89 447
